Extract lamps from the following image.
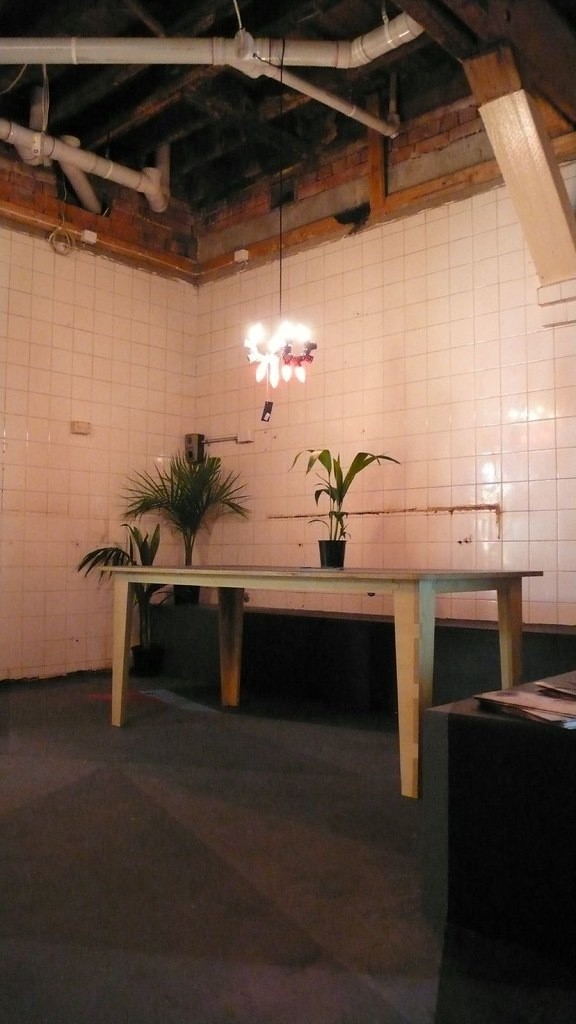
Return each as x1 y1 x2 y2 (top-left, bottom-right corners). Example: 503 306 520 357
245 36 319 388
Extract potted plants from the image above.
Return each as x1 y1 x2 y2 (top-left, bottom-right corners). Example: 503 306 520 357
77 523 173 675
116 448 254 605
288 448 402 570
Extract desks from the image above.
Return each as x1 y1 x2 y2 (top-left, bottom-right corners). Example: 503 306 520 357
97 569 543 799
425 672 576 967
146 601 576 707
